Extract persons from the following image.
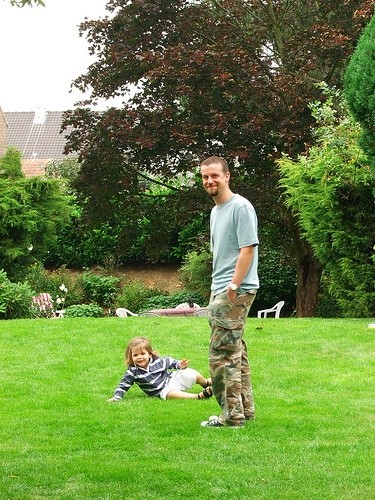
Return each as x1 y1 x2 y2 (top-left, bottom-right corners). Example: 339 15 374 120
199 157 260 429
107 336 214 403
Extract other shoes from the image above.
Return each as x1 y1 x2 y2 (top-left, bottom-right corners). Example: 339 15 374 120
209 416 249 422
201 421 245 428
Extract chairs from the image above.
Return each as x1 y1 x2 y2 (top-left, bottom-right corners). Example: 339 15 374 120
175 303 200 313
258 300 284 318
28 293 65 319
137 311 162 317
115 308 139 317
193 308 209 316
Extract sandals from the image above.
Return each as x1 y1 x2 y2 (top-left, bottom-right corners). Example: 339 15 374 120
202 378 211 389
198 385 213 399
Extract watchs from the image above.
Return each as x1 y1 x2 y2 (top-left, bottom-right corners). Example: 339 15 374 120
228 283 238 291
